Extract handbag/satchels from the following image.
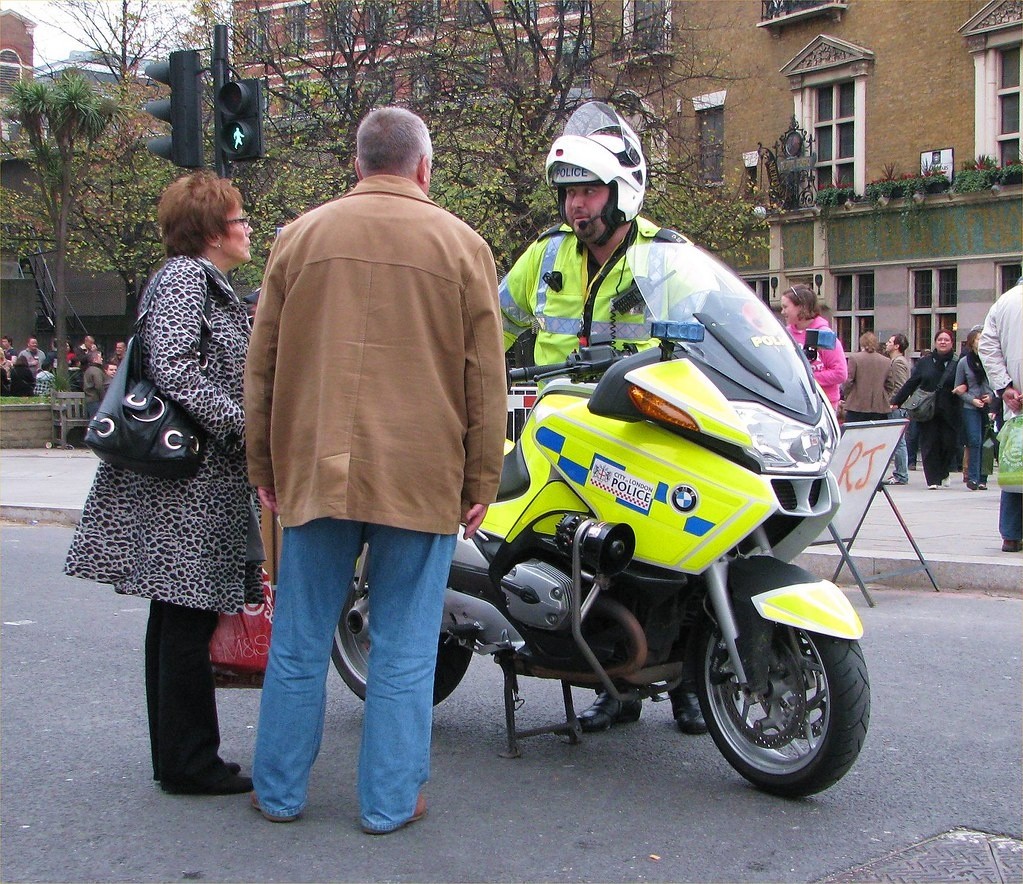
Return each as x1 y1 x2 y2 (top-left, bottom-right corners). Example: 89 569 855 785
84 262 213 478
900 388 936 422
995 404 1023 493
208 568 275 689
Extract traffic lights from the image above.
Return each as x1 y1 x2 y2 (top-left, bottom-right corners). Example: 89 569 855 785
145 51 204 167
243 287 262 324
218 77 264 162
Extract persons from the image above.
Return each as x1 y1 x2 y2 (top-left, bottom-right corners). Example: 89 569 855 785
245 104 507 833
496 101 719 733
780 284 847 414
0 332 126 417
840 326 1005 491
977 275 1023 552
62 173 265 796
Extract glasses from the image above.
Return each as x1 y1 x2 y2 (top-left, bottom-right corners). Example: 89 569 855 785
225 215 251 229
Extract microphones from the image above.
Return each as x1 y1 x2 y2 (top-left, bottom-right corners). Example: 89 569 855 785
579 213 604 230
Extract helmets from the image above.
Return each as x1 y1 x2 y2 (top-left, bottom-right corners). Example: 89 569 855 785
544 133 647 230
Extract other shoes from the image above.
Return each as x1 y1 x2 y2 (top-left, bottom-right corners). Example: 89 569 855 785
361 793 425 834
909 463 916 470
250 790 296 821
225 763 240 775
165 775 254 795
979 482 987 490
1002 540 1023 551
928 484 938 488
882 476 908 485
942 475 951 487
966 480 977 490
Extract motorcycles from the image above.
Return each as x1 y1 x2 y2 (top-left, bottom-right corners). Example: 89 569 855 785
330 242 871 797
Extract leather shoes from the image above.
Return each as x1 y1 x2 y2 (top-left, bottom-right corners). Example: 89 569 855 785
668 690 707 734
564 685 642 732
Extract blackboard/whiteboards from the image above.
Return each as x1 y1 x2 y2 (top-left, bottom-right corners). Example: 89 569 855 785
800 419 911 545
